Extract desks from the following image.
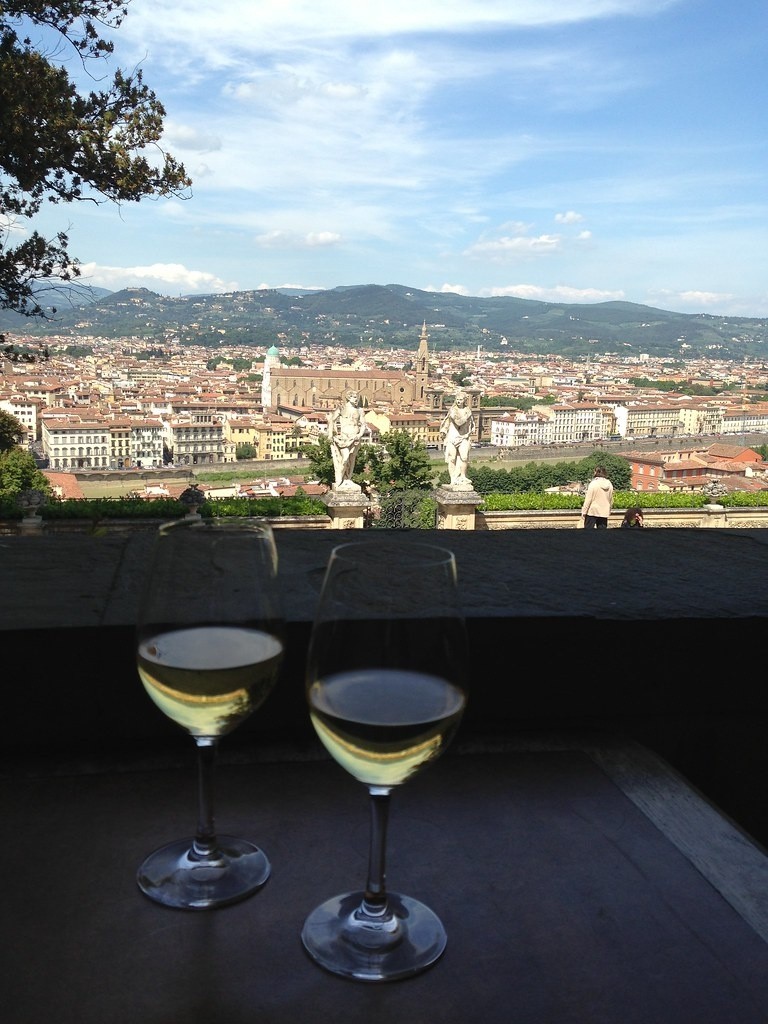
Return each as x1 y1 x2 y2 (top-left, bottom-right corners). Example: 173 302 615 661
0 718 768 1024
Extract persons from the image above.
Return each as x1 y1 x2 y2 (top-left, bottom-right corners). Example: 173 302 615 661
582 466 613 529
328 391 366 487
440 392 475 484
622 507 644 528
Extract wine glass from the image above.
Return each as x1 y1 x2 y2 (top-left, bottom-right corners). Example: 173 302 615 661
134 518 289 913
301 546 472 985
